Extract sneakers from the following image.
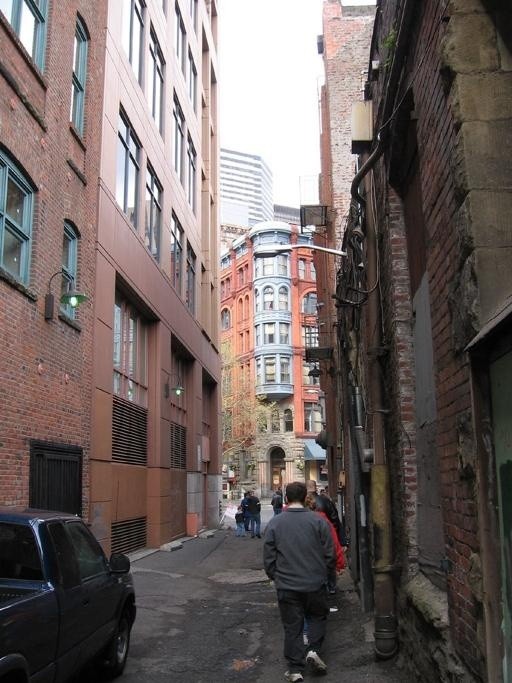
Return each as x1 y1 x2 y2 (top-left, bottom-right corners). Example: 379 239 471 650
305 650 328 674
250 534 262 539
284 670 304 682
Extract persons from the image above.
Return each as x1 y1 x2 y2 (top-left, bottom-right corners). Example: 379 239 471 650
234 480 347 682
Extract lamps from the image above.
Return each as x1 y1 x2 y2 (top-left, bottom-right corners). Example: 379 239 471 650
165 373 185 399
44 270 88 320
307 356 337 385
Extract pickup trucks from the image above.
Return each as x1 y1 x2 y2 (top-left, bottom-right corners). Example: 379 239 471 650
1 505 138 682
